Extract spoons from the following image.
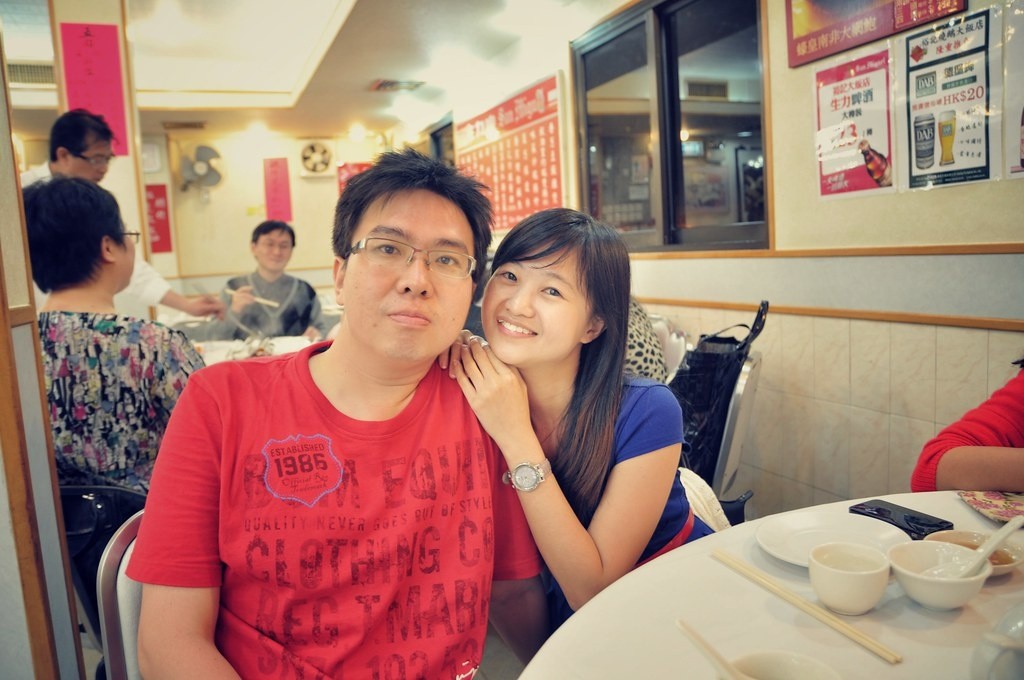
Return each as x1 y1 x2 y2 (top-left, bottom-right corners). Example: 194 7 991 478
918 513 1024 577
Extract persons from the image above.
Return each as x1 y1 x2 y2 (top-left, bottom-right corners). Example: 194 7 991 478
438 207 717 637
213 220 328 343
20 108 228 323
905 359 1024 494
123 148 557 679
22 171 210 497
621 295 670 383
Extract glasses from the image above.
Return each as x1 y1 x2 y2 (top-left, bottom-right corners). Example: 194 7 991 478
346 237 477 278
116 231 140 244
73 152 118 170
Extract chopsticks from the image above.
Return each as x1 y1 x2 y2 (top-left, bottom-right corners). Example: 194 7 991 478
193 284 255 339
224 287 279 309
669 613 751 679
711 549 902 664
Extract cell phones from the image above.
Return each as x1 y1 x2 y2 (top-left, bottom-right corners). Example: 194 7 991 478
848 498 954 541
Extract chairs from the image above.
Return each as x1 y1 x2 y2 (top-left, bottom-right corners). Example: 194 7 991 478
59 483 145 656
711 353 763 525
95 509 144 680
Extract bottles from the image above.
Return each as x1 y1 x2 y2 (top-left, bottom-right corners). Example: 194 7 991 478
858 141 892 187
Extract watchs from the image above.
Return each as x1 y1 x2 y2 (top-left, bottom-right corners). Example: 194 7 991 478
502 458 552 493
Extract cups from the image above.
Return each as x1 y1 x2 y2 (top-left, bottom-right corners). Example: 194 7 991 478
938 111 956 166
914 113 935 169
809 543 891 616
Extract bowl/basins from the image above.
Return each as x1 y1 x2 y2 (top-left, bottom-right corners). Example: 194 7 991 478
722 653 832 680
888 541 993 613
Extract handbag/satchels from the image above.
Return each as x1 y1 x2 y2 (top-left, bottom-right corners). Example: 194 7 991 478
58 485 147 583
718 490 754 526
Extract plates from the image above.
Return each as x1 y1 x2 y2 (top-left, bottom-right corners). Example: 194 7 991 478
187 337 311 366
754 508 914 569
924 529 1024 576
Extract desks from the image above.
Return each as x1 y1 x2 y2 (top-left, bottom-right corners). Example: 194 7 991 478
192 335 313 367
517 492 1024 680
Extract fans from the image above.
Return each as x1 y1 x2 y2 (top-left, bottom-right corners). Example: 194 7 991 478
172 142 230 193
